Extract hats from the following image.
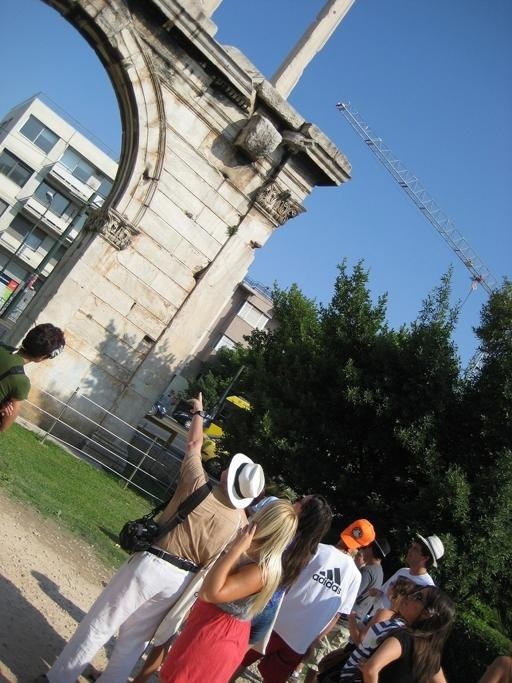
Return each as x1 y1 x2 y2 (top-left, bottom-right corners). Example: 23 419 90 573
339 518 376 550
415 532 445 569
373 535 392 557
226 451 266 510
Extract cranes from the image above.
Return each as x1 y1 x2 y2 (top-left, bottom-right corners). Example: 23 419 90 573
331 94 506 300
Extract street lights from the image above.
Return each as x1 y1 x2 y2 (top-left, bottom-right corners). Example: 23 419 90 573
0 188 55 279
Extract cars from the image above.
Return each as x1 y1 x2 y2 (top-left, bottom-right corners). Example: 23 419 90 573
144 399 218 431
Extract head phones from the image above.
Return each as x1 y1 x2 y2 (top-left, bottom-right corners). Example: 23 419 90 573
51 344 64 358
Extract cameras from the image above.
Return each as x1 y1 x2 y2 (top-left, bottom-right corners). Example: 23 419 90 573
129 518 157 541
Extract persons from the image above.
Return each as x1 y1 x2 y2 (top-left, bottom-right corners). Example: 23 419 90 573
36 390 512 683
1 321 67 432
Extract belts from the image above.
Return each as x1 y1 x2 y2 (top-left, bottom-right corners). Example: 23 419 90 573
148 546 199 573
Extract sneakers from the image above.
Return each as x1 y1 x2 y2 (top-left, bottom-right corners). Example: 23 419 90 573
291 670 303 678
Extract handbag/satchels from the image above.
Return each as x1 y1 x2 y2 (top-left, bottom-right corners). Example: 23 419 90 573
317 643 356 682
118 515 163 556
